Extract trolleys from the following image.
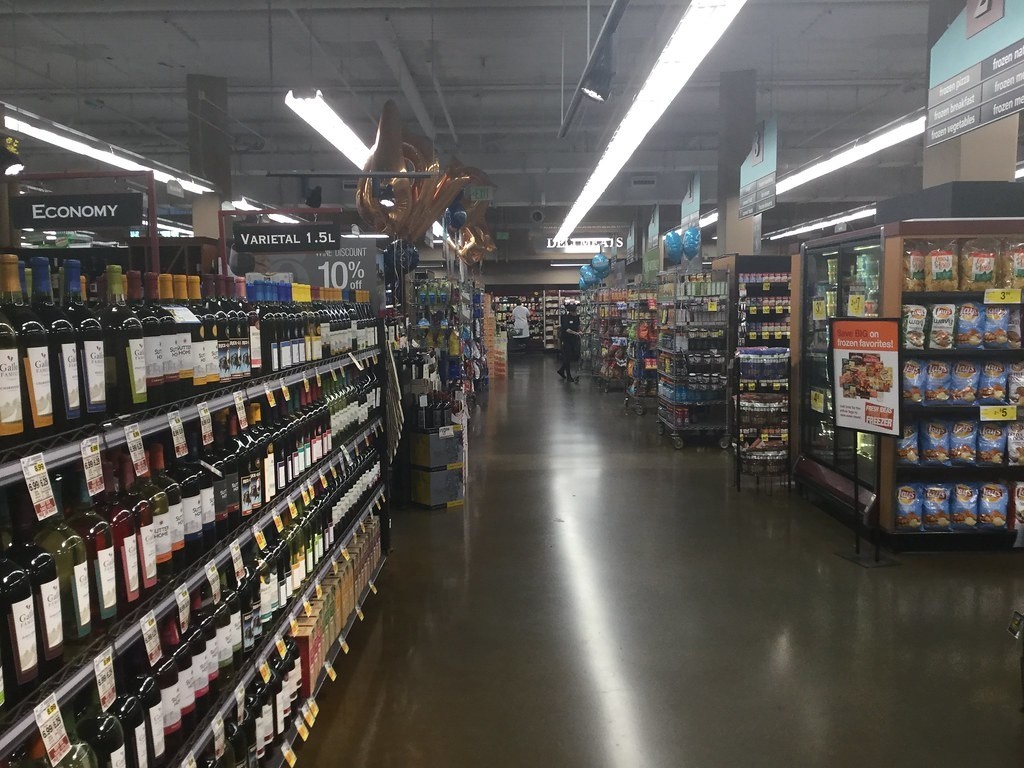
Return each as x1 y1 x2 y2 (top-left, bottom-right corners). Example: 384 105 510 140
574 333 599 384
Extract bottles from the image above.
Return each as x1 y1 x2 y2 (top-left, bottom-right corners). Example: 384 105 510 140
386 279 463 430
0 254 381 768
658 332 690 427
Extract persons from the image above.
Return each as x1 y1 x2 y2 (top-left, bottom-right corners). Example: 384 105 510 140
512 299 531 347
557 304 583 382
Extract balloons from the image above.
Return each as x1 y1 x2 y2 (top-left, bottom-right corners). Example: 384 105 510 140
381 238 419 282
683 226 701 261
445 202 467 229
592 253 609 272
578 265 610 291
665 231 683 265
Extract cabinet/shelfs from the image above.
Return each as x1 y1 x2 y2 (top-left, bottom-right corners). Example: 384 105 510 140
0 211 397 768
407 212 1024 553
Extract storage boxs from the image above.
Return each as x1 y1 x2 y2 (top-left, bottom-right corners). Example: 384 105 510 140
285 516 380 699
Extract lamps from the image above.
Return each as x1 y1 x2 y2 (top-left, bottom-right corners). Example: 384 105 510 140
306 185 321 208
284 86 374 168
552 0 747 243
0 102 302 226
381 184 397 210
653 104 927 243
579 46 613 104
764 203 879 242
